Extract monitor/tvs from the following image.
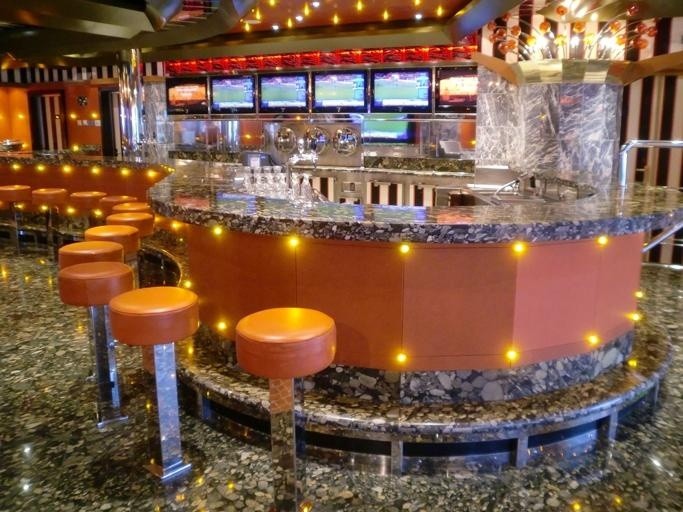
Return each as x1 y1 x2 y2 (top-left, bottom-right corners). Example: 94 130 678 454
345 97 414 143
435 67 478 112
370 67 432 113
311 69 367 113
166 76 208 115
258 72 308 113
209 75 256 114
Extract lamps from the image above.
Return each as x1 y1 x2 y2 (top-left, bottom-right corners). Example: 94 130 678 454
488 5 657 62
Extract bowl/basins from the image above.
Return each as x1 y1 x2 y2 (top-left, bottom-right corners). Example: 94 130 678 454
0 140 26 151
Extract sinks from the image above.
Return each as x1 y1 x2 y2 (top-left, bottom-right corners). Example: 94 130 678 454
480 192 520 197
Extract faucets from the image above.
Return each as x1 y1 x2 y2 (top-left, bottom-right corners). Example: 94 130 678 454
287 137 319 187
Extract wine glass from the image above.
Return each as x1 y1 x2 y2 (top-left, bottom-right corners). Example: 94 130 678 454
230 166 314 201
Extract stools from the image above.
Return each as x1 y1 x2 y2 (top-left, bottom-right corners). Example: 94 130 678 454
57 262 134 429
109 285 201 488
236 308 336 512
0 184 137 260
58 202 154 384
